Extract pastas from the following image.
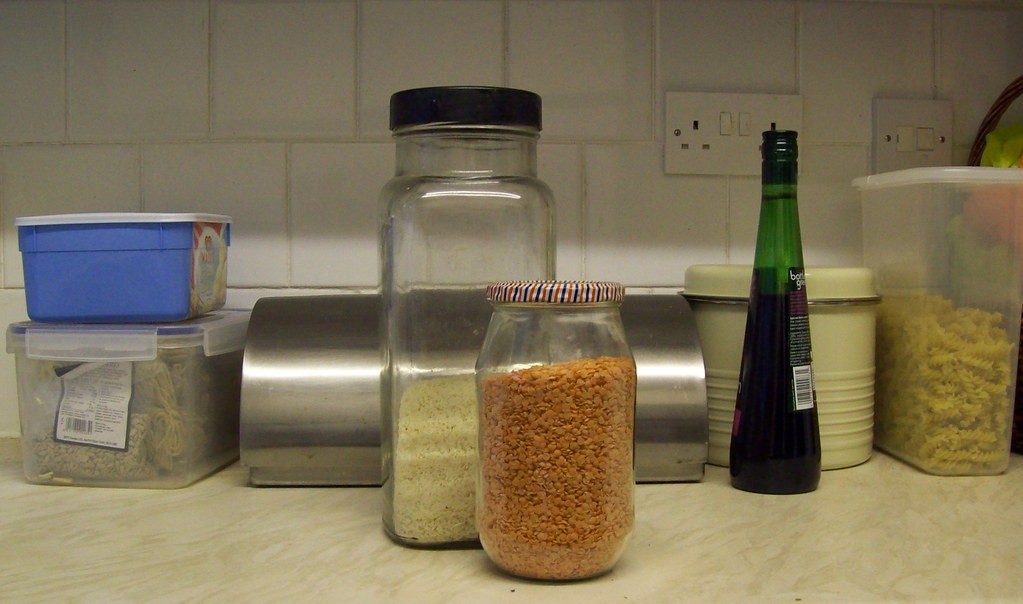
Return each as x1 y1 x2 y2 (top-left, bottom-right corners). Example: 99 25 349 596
871 293 1014 472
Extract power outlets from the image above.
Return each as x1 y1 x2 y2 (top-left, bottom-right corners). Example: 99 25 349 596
663 90 803 176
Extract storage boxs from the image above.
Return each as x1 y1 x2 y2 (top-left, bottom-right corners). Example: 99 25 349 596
6 307 251 488
851 164 1023 477
15 211 232 324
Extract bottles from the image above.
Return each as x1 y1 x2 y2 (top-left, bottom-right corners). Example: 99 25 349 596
473 280 638 582
377 85 556 549
728 129 823 495
